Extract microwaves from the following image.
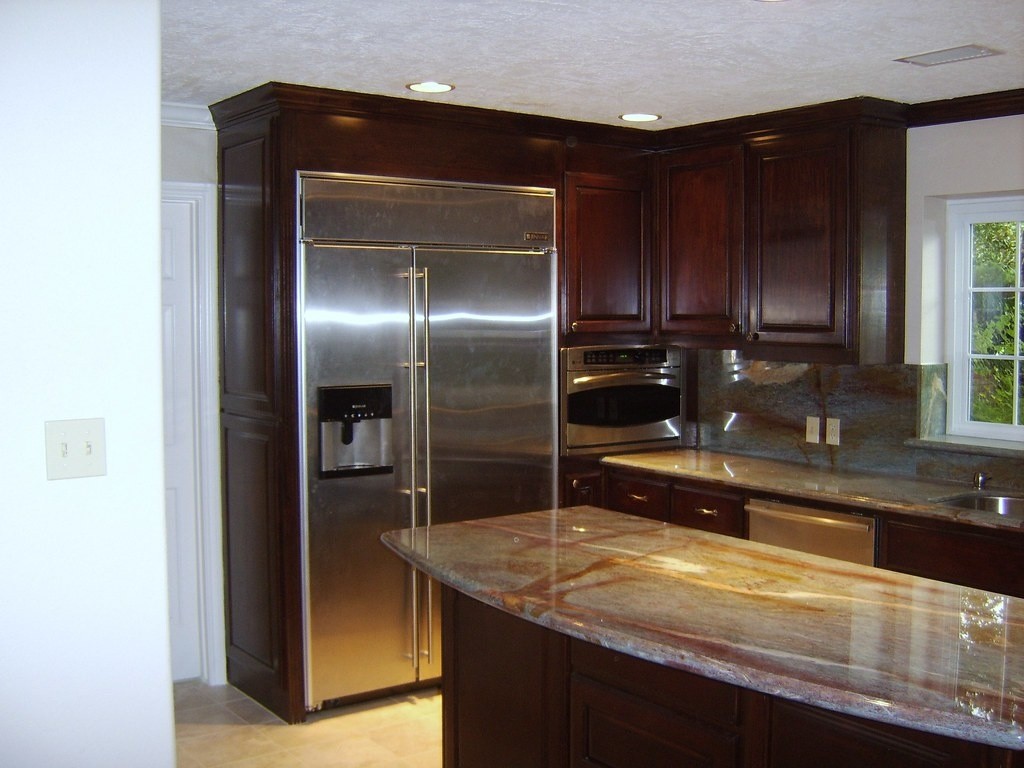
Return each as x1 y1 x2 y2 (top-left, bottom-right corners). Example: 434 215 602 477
563 342 693 454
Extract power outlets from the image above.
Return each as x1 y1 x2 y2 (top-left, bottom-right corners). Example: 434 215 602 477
826 419 840 447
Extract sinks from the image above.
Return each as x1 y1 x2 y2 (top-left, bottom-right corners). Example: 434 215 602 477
928 491 1024 520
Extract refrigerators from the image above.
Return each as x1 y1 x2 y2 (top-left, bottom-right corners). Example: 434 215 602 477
289 170 564 716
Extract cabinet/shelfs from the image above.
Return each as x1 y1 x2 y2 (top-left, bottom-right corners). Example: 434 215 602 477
604 471 743 537
653 118 908 365
438 584 998 768
888 520 1024 598
562 172 653 336
